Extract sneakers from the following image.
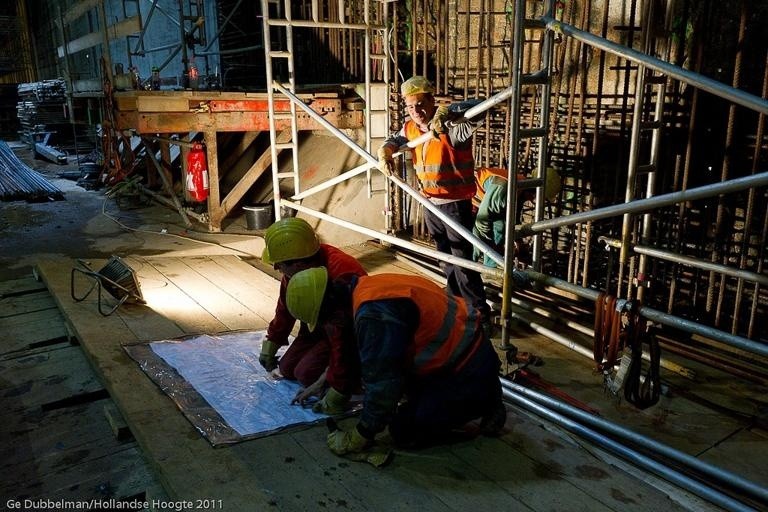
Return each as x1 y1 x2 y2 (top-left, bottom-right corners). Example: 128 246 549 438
478 399 507 436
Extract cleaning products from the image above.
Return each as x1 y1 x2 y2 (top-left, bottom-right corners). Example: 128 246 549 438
188 53 199 90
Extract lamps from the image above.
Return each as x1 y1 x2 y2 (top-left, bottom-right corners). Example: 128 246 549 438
71 254 147 317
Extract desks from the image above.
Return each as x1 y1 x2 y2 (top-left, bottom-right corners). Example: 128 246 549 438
114 90 366 235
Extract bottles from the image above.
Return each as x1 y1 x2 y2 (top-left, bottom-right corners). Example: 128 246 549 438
150 63 159 90
187 58 200 91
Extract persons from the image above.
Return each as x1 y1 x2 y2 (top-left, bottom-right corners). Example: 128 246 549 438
260 218 370 387
287 266 507 454
377 76 496 336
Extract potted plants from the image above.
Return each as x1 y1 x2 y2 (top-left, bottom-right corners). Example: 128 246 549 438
104 174 144 210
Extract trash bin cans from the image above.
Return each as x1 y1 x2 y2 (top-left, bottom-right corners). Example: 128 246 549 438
84 162 100 191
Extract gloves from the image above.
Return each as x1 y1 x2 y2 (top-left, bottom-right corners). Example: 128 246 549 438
427 105 450 139
378 148 396 176
328 425 367 455
259 340 280 370
312 387 352 415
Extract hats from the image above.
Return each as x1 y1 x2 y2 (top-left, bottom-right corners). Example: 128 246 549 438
260 218 321 264
531 166 561 204
398 76 435 98
286 266 328 332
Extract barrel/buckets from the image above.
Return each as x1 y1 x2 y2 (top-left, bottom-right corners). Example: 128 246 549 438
242 203 271 230
